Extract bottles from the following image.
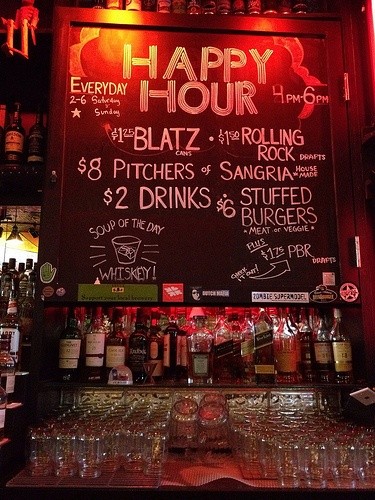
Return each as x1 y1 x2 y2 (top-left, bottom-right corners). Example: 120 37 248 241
0 384 8 440
0 258 40 372
57 306 353 388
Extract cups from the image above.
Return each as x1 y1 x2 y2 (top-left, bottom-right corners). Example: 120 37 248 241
29 403 168 477
170 393 239 448
224 405 375 483
